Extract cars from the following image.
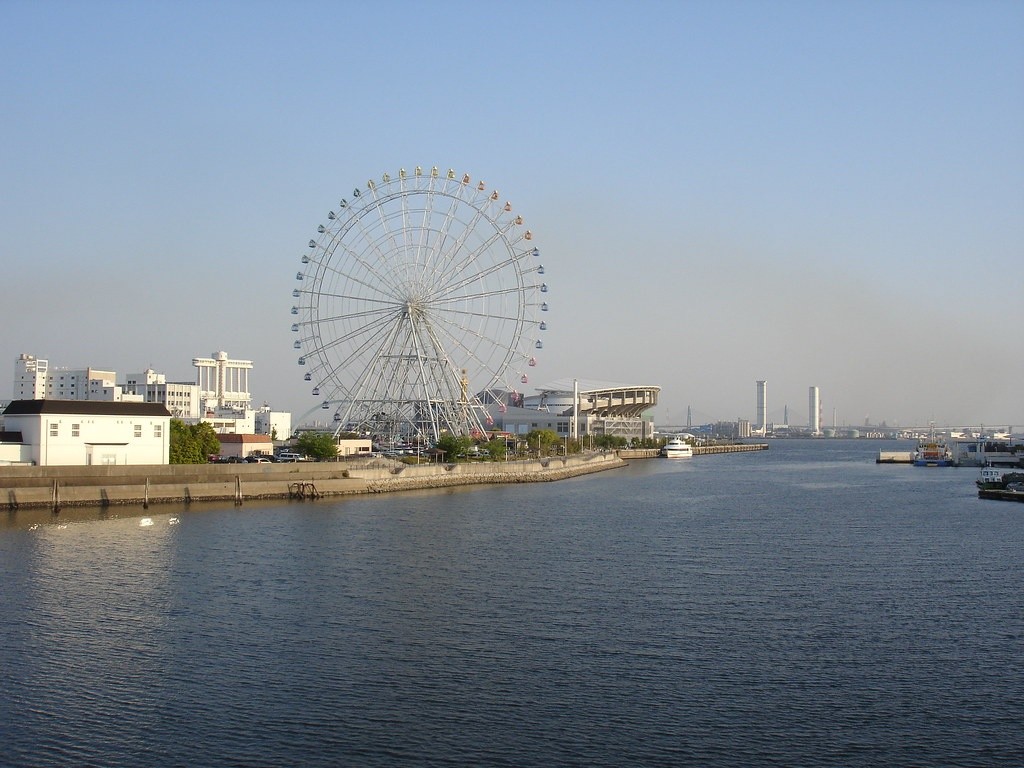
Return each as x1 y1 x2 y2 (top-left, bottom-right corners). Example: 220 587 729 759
205 452 333 464
372 448 493 460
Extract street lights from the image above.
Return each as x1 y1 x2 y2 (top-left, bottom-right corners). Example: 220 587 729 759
336 433 341 462
579 434 584 454
416 433 421 464
504 434 508 461
564 435 568 455
538 435 541 459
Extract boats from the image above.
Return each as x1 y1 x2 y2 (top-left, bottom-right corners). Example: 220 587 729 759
979 460 1003 483
661 435 694 458
911 421 953 468
